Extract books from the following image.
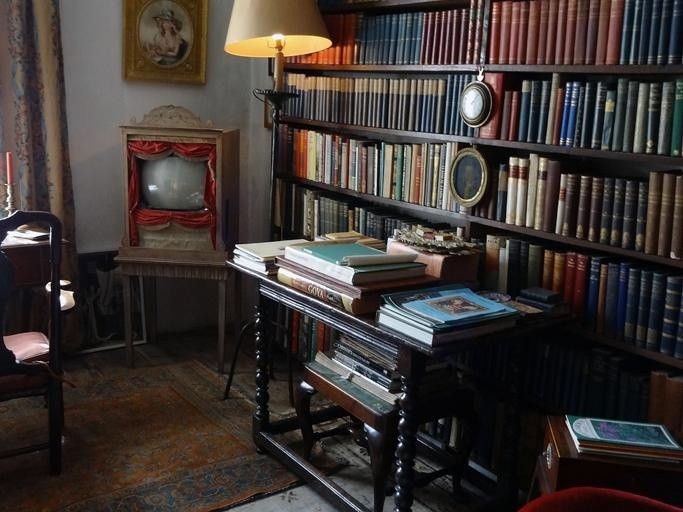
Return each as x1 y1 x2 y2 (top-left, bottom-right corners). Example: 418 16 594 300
272 304 340 363
275 178 465 242
566 413 683 464
377 283 521 347
474 153 683 260
281 72 476 136
313 333 505 406
386 237 483 292
234 239 312 275
276 242 440 316
276 124 474 213
524 334 683 443
8 224 49 240
479 73 683 156
314 230 386 251
284 0 683 64
484 233 683 360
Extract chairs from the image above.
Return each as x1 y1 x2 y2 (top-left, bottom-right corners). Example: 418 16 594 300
0 209 63 475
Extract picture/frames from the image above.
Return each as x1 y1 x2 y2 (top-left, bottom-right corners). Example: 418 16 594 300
448 147 488 208
120 0 207 84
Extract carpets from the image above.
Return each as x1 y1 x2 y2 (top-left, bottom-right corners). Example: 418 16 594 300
1 340 352 510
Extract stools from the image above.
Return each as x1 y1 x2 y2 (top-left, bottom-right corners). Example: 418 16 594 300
298 360 473 511
525 415 681 506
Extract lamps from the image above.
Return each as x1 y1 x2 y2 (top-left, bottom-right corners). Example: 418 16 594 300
223 0 333 406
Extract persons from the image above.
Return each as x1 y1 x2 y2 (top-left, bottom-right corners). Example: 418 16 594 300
147 8 185 66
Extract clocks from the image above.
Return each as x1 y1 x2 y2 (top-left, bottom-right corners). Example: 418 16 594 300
458 81 493 128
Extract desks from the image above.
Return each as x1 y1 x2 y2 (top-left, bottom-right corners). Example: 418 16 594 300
2 228 69 331
224 236 576 512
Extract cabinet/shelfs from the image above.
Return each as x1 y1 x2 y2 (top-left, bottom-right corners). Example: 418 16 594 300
269 0 680 431
